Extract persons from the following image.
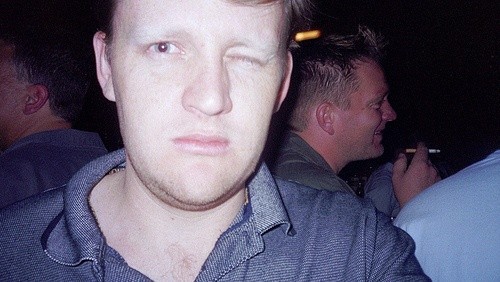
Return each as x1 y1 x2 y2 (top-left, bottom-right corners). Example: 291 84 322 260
0 0 431 282
269 29 440 217
0 0 109 207
393 149 500 282
364 163 399 219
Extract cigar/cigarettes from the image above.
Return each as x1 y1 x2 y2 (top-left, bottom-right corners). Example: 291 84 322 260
406 149 440 154
